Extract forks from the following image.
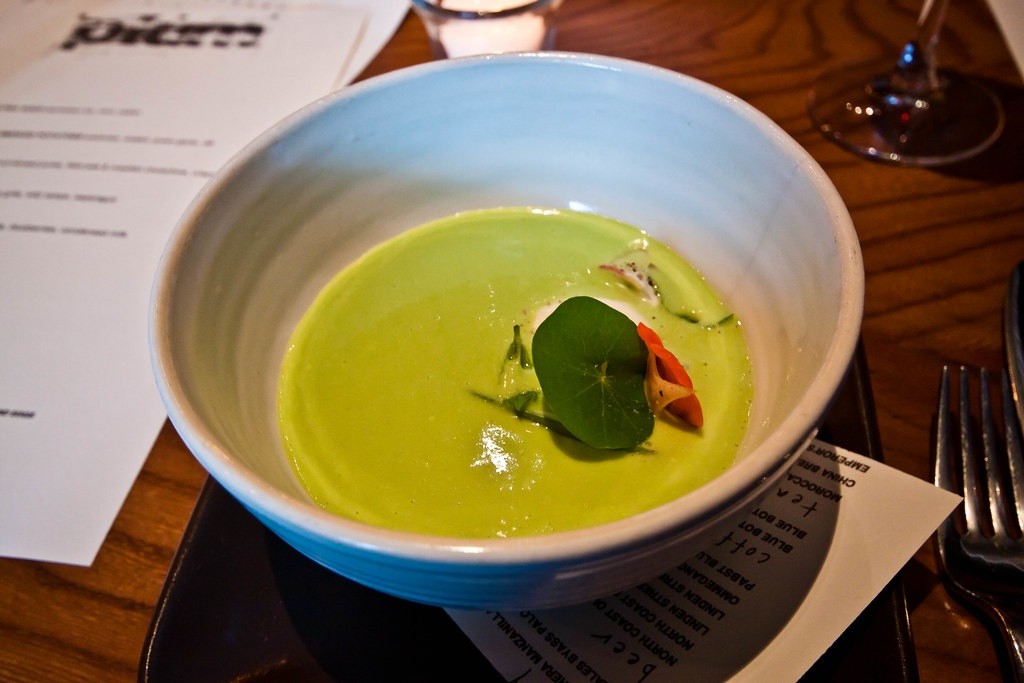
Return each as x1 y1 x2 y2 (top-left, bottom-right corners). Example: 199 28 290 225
928 364 1024 683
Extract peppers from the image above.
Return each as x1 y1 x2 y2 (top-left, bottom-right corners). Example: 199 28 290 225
637 322 704 428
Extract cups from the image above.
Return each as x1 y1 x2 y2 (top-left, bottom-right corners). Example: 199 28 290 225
408 0 563 56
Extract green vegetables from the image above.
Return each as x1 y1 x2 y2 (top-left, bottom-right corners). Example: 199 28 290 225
502 295 655 451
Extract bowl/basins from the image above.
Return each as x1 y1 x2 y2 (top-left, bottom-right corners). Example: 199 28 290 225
145 53 863 614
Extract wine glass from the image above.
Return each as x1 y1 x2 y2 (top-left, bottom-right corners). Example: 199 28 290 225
807 0 1005 168
